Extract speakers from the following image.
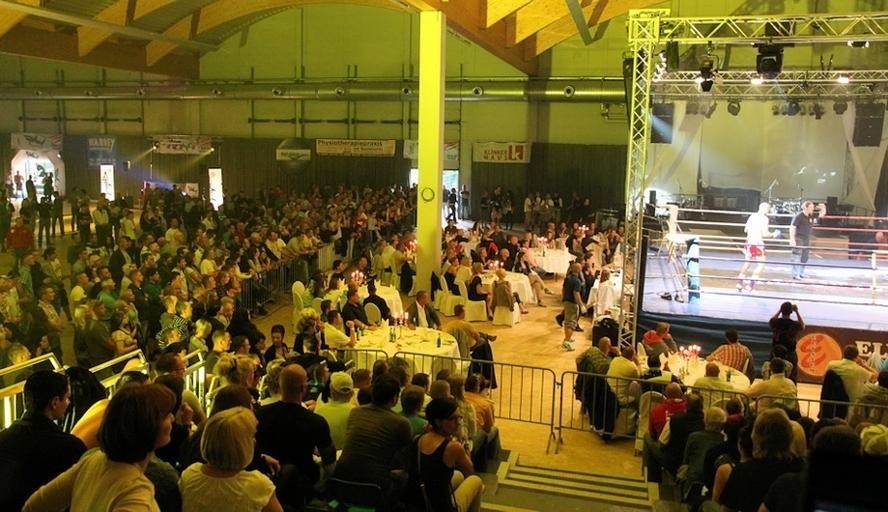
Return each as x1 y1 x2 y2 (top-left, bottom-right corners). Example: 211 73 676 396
651 104 673 143
852 105 885 146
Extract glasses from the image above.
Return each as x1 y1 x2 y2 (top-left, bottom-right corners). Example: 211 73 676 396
444 413 464 422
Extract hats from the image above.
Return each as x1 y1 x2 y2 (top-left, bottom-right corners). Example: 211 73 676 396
330 371 354 394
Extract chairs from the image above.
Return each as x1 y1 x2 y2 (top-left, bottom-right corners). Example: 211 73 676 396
325 477 382 512
368 248 416 290
637 342 645 358
817 369 850 419
456 279 488 322
711 398 732 411
292 281 305 299
774 392 800 412
440 275 465 316
429 271 442 310
572 359 636 446
493 285 521 328
292 291 318 335
635 393 671 456
311 297 323 313
742 358 750 375
446 327 475 376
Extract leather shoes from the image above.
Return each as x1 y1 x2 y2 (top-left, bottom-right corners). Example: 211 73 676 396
574 325 585 333
555 315 564 327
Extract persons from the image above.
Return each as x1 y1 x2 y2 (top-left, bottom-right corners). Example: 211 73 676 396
65 344 211 461
316 371 357 449
205 284 495 401
761 346 793 380
744 357 799 410
398 370 495 466
544 188 627 353
254 365 336 507
303 257 420 307
710 424 756 501
788 199 828 282
754 425 863 512
0 163 142 370
136 180 214 358
189 258 245 322
661 407 727 499
432 185 556 321
180 406 286 512
142 446 183 512
574 337 621 376
733 202 782 293
647 380 686 440
635 352 686 395
701 409 806 511
824 344 880 420
853 424 887 511
209 183 436 265
603 344 641 417
324 375 418 508
640 319 680 360
69 369 152 449
0 370 89 512
642 394 703 481
411 398 483 511
766 300 805 389
706 327 755 375
184 385 282 483
26 382 176 512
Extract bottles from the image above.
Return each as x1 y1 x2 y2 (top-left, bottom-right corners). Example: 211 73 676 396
437 333 441 348
357 327 360 341
393 329 396 342
389 328 393 340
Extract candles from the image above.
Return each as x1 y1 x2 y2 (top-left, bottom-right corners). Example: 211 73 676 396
489 260 504 273
350 270 363 281
409 239 417 251
679 344 701 361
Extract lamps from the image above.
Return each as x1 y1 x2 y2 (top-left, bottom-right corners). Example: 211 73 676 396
751 76 762 86
839 73 850 86
685 96 848 120
153 141 159 151
642 95 652 117
753 43 794 80
701 44 713 92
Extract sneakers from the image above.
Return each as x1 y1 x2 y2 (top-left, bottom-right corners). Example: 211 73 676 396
735 278 745 292
561 343 576 352
568 335 576 342
744 279 754 292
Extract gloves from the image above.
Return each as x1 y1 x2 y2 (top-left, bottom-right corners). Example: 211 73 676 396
773 228 782 240
